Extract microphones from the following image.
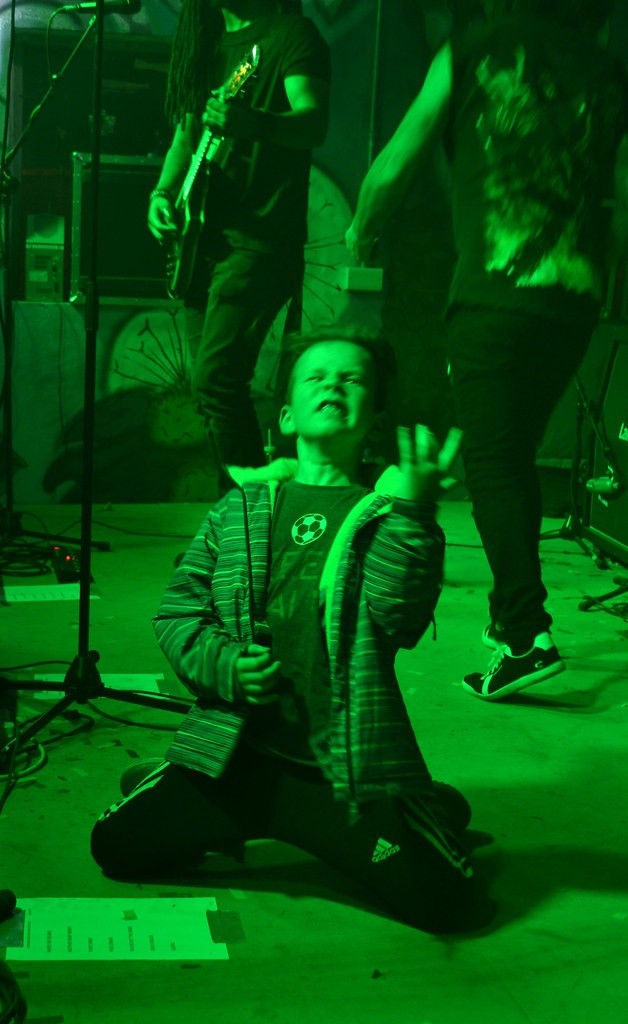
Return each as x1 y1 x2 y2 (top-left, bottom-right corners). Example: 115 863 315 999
585 477 621 495
57 0 142 14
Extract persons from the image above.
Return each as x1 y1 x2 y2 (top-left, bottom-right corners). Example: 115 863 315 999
345 1 616 701
88 330 485 935
147 0 331 566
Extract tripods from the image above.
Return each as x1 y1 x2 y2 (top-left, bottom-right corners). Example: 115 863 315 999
0 0 195 762
540 337 621 569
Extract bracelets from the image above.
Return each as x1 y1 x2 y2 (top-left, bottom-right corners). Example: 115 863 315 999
150 189 175 202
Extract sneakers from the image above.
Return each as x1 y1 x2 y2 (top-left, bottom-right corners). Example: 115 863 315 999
119 757 168 798
461 632 566 702
429 780 472 832
482 624 504 650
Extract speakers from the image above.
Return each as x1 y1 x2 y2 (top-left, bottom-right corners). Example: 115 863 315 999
582 318 628 565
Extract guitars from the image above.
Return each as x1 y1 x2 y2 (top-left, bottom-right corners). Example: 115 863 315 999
156 43 265 299
